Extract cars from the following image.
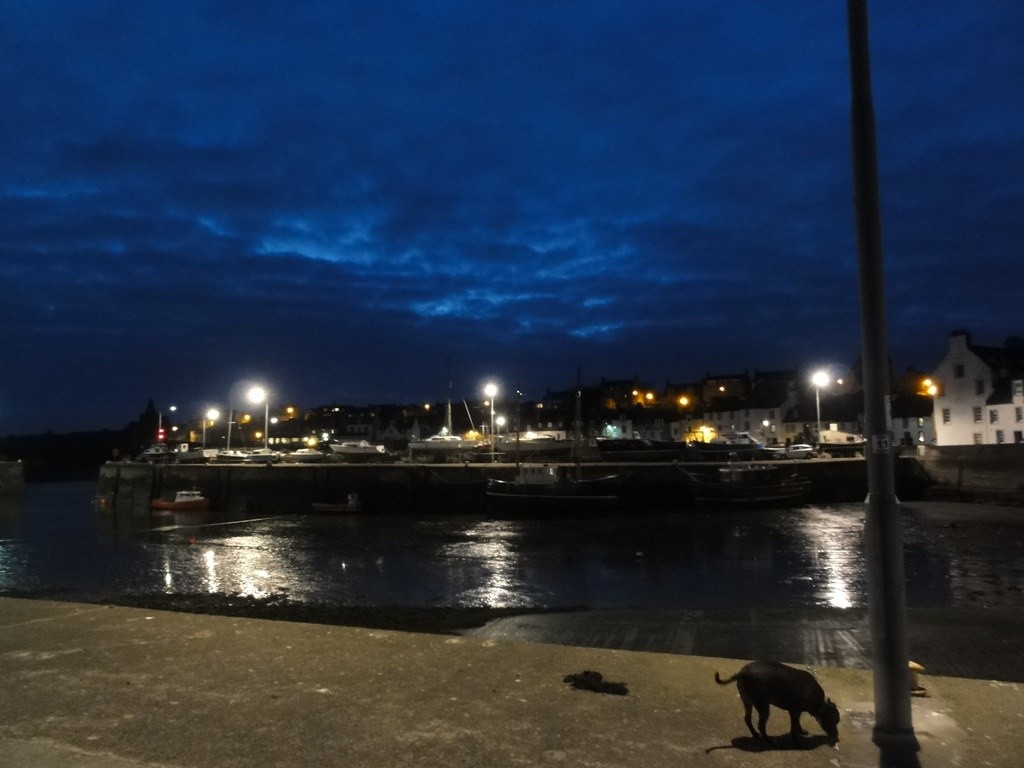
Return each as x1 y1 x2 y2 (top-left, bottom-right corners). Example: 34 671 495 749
777 443 816 461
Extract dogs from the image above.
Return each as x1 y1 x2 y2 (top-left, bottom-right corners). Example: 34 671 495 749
714 659 841 738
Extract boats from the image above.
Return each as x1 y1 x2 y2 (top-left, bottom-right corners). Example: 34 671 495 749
313 494 373 513
674 463 815 508
818 429 868 454
482 469 625 513
140 382 763 463
148 490 210 513
